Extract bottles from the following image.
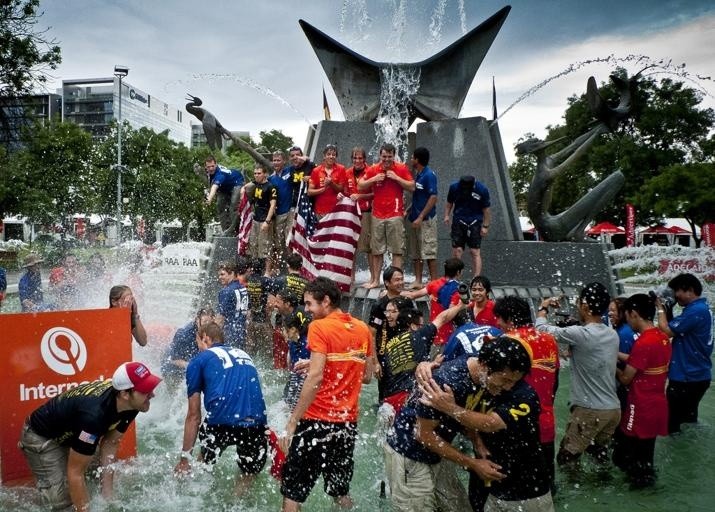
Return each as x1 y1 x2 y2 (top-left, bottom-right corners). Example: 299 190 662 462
457 283 471 305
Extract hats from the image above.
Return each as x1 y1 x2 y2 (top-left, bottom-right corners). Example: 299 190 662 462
23 254 44 268
112 362 163 394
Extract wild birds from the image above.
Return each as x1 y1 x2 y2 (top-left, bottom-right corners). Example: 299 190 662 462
183 92 224 153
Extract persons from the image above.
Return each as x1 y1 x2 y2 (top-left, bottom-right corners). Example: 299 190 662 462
204 144 490 290
0 251 715 511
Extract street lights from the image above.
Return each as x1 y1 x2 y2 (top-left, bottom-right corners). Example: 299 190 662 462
114 63 132 246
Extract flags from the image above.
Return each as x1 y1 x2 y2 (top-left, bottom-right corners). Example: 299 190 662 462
322 90 331 121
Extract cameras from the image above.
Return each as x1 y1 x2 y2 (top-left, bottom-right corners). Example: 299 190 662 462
660 296 675 311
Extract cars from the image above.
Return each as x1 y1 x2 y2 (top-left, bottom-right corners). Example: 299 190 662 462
33 229 85 248
